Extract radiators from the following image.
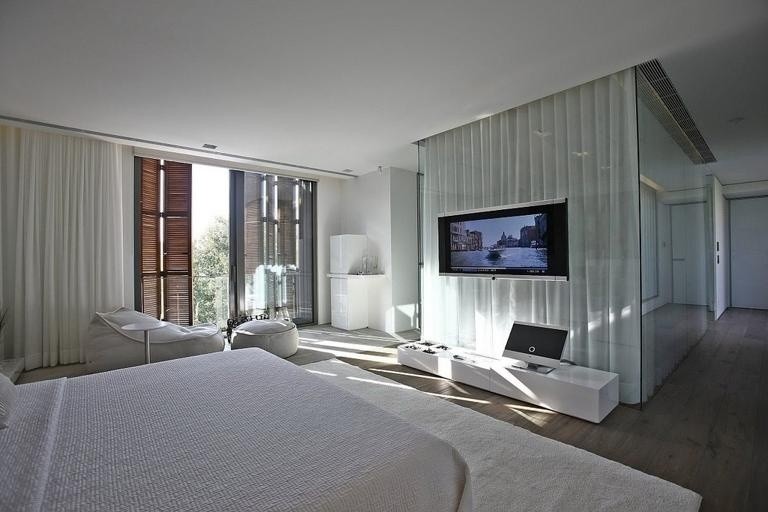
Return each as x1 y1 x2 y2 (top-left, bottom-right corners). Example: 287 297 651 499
254 263 299 322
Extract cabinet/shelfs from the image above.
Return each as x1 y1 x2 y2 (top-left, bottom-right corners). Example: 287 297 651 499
328 273 369 331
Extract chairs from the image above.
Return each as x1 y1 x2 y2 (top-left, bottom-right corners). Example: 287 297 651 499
84 306 225 373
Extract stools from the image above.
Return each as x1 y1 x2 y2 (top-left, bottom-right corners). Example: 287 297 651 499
230 318 299 359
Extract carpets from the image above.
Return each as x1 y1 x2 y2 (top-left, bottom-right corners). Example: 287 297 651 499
283 348 336 366
300 356 704 512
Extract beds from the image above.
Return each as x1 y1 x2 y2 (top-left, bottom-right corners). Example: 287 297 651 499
0 346 473 512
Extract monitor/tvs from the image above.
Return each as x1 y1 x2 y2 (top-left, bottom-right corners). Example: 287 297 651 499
436 197 569 282
502 321 569 374
